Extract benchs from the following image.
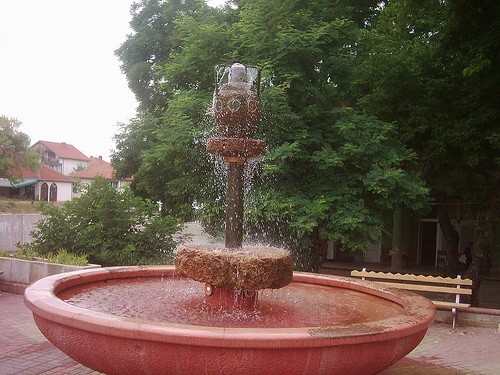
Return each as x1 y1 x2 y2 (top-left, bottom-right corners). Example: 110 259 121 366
351 267 473 327
